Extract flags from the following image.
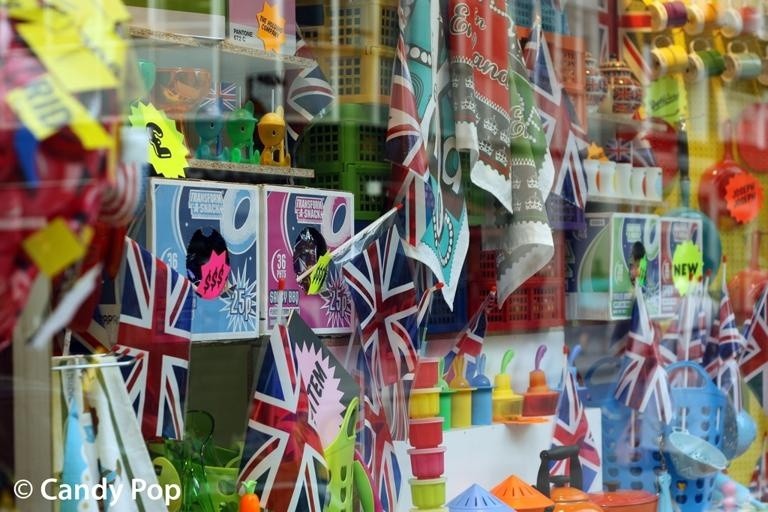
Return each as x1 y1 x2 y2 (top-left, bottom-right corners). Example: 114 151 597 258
0 0 768 511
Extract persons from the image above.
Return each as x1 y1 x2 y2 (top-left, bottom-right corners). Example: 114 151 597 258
0 0 768 511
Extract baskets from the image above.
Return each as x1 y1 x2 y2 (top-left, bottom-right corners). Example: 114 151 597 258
579 356 726 512
424 227 566 331
289 104 387 218
